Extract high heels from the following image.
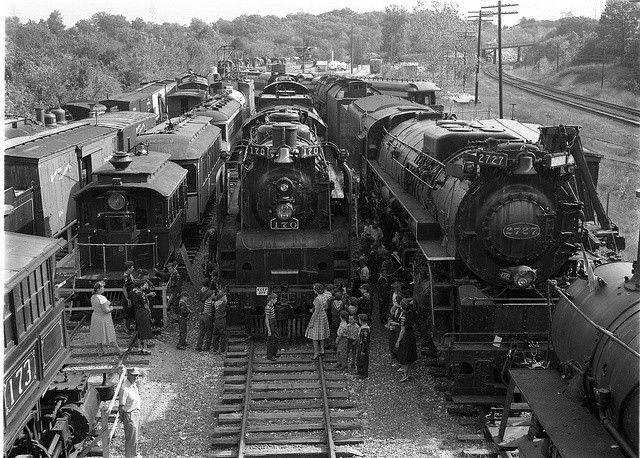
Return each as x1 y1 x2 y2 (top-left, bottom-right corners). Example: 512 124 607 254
143 349 151 354
138 348 142 353
397 368 404 371
399 376 409 382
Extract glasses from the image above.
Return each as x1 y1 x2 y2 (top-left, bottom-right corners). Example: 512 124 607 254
131 375 138 377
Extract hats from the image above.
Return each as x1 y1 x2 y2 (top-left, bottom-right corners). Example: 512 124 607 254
210 270 218 276
141 269 150 275
166 263 174 267
360 284 370 289
218 292 227 299
201 287 209 293
177 291 190 297
124 368 143 376
207 290 216 297
358 313 368 322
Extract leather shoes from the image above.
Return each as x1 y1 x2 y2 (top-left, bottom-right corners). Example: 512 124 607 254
128 328 133 332
221 349 227 355
268 357 277 361
274 355 280 358
312 351 319 360
205 347 211 351
170 320 178 323
196 348 203 352
319 351 324 355
124 330 130 335
355 372 361 375
358 376 367 379
177 346 186 349
184 343 190 346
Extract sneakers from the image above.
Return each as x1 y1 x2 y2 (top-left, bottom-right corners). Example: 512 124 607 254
334 364 341 368
213 349 219 355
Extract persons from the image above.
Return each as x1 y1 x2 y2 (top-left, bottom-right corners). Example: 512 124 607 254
328 219 416 384
153 261 182 324
89 281 129 356
118 368 141 458
264 293 280 361
136 269 156 309
176 292 190 350
196 278 228 355
304 282 331 360
123 261 135 334
133 279 152 354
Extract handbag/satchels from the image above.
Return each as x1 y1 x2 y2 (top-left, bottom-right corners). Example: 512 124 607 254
149 313 155 325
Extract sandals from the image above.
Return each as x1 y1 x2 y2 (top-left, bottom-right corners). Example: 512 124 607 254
99 350 107 356
119 348 129 356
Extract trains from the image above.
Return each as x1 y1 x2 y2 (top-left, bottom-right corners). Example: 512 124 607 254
310 73 640 458
220 66 357 318
3 210 104 446
